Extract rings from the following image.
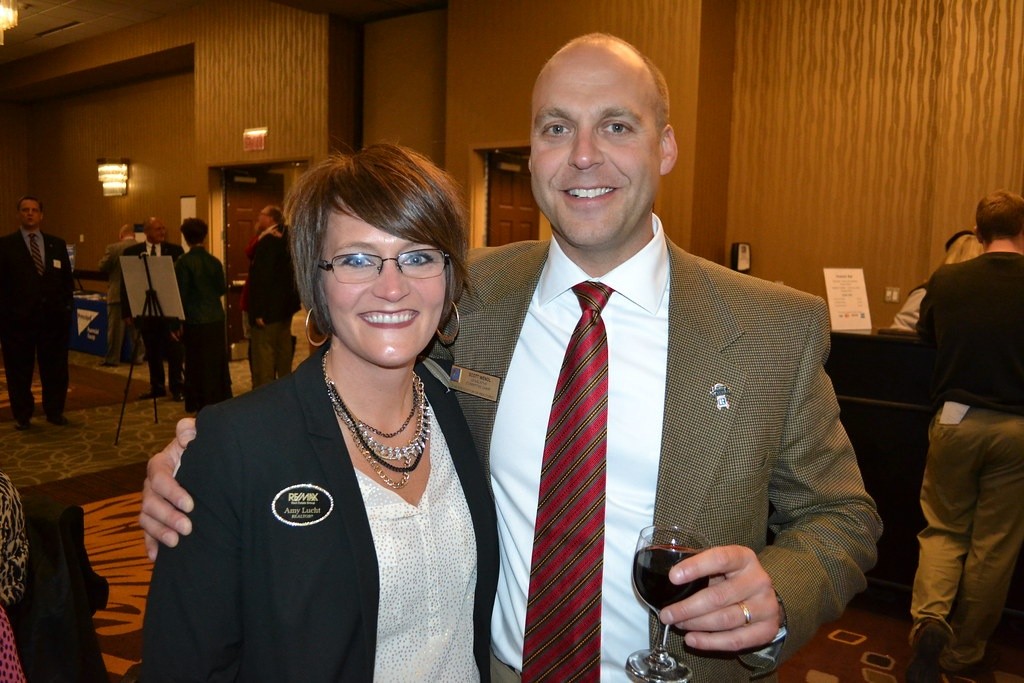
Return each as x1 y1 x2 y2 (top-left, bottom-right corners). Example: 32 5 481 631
738 601 751 624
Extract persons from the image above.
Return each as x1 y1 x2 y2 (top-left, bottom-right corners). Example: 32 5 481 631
890 230 984 331
120 219 183 402
0 194 75 429
176 217 232 413
0 469 28 683
140 142 500 683
245 207 301 388
139 33 885 683
906 189 1024 683
98 225 143 366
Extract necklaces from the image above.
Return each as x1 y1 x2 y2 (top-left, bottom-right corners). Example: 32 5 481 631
323 350 430 488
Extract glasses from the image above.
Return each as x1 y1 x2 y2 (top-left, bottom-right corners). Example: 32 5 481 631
317 248 451 285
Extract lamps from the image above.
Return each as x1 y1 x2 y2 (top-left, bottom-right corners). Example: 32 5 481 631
0 0 18 45
96 158 128 196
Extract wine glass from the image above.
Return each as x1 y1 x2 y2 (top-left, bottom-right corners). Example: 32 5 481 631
625 526 711 683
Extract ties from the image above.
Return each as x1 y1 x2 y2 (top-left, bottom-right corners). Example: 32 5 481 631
150 244 156 256
28 233 44 278
521 281 614 683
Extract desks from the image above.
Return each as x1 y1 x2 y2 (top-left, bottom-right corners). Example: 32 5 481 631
68 290 130 361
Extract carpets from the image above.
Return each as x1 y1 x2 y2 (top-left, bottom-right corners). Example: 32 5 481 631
17 459 1024 683
0 354 171 424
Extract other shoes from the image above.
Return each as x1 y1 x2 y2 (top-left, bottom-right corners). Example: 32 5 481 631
138 388 167 400
173 391 185 402
905 619 949 683
14 419 30 431
938 643 1000 675
47 415 68 426
